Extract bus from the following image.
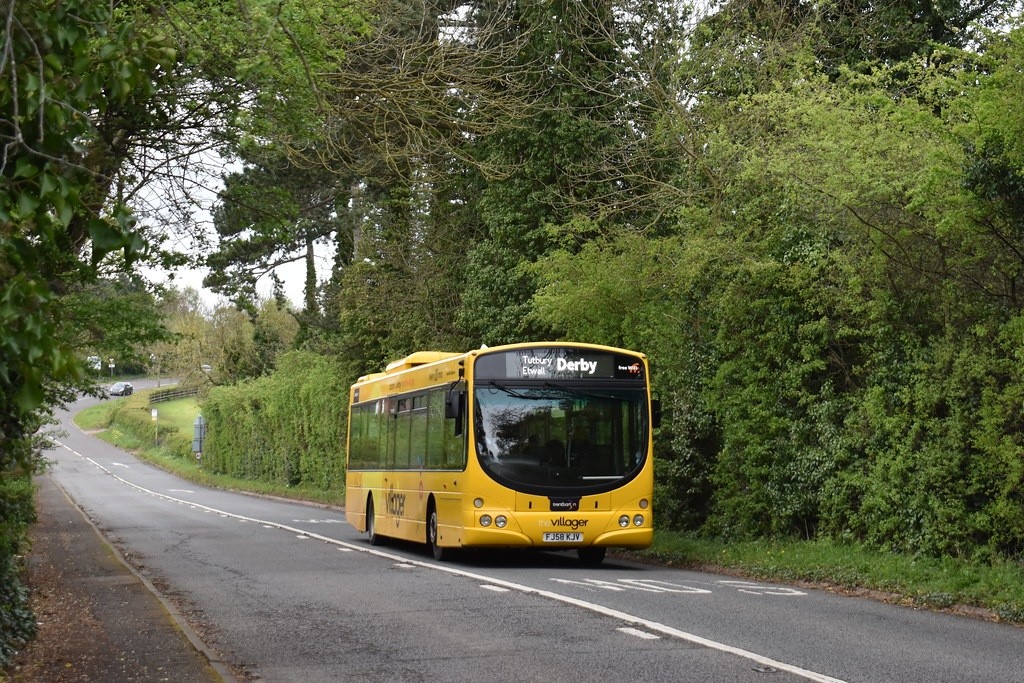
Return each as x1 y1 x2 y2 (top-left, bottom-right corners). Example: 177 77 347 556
346 340 654 571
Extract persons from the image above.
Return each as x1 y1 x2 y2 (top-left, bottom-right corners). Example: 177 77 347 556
566 426 593 474
521 434 543 457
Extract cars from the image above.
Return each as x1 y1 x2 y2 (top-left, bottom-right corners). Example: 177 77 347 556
110 382 133 397
